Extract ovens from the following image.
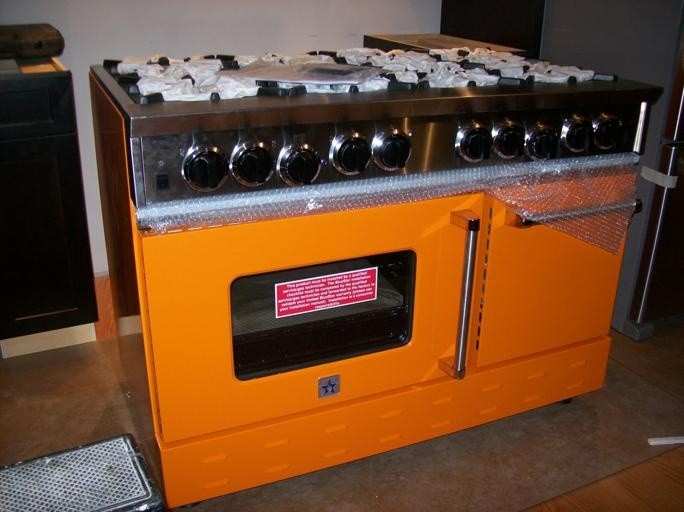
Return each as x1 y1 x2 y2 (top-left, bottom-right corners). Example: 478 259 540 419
103 151 646 510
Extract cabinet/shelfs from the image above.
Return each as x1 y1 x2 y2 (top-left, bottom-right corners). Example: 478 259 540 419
1 56 99 339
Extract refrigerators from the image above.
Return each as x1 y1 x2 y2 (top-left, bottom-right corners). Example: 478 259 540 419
538 0 684 341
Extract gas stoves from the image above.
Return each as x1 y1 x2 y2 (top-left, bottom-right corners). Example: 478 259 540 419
89 50 664 208
